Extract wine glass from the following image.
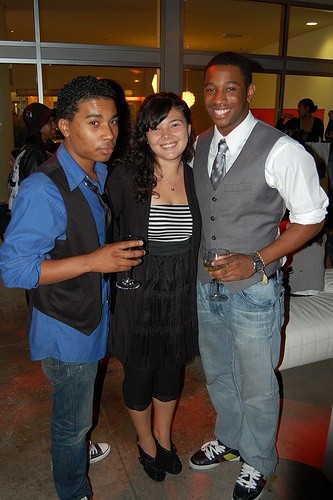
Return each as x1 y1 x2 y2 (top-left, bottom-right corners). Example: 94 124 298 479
116 235 144 290
203 248 230 302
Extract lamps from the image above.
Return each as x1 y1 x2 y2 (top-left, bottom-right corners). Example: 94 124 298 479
182 68 196 109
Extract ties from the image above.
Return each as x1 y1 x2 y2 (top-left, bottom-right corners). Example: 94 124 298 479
84 178 117 313
210 139 229 192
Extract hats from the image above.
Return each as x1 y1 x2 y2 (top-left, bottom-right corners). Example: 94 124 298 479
23 102 54 139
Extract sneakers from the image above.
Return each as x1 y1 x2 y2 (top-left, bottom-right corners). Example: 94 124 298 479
189 439 240 470
232 459 269 500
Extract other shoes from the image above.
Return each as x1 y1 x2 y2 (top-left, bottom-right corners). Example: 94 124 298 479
88 441 111 464
136 433 182 482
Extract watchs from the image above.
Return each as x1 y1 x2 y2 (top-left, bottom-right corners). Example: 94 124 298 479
247 252 264 273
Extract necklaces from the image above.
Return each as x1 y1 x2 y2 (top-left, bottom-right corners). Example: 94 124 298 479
156 167 182 192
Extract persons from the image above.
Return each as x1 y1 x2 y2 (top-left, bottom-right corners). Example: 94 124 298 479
188 51 329 500
325 110 333 168
111 91 202 482
280 220 327 296
9 148 21 170
276 97 325 143
0 75 146 500
7 102 57 307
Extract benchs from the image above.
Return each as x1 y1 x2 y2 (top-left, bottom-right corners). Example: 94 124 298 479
276 267 333 371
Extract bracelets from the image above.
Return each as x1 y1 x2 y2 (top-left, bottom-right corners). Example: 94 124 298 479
255 250 269 285
